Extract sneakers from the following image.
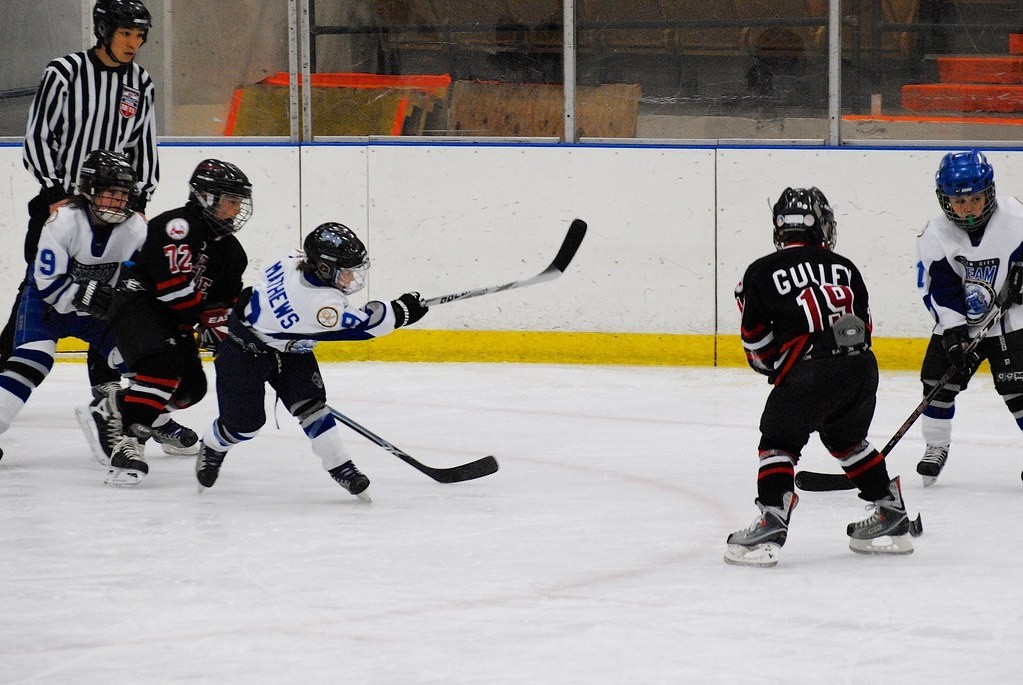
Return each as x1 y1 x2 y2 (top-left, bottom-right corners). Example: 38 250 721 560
847 475 922 554
76 390 123 465
329 460 372 503
916 443 950 487
151 419 201 455
724 491 799 567
91 381 122 398
195 437 228 493
105 423 154 487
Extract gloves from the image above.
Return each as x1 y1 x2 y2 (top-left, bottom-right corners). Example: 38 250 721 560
942 325 979 379
1009 262 1023 305
197 306 230 345
72 278 113 318
391 292 428 329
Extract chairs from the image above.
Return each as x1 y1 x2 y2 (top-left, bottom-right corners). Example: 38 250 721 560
371 0 930 108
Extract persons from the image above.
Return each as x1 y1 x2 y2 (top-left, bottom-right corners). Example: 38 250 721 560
0 150 202 464
196 222 430 505
723 187 914 569
74 159 253 484
0 0 159 400
915 149 1023 488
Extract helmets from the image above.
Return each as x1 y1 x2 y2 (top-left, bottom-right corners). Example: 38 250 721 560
78 150 138 224
772 187 837 250
304 222 367 285
189 158 253 237
93 0 152 49
935 150 996 232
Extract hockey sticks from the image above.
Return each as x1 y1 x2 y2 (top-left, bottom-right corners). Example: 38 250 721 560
794 295 1014 493
908 512 924 537
419 218 588 307
324 403 498 483
